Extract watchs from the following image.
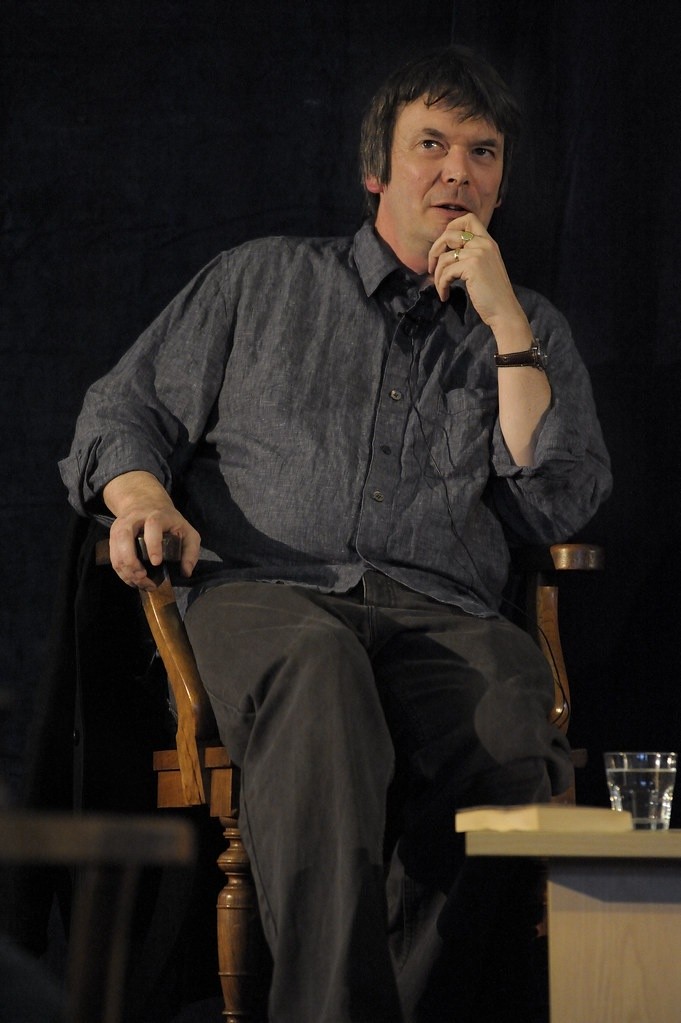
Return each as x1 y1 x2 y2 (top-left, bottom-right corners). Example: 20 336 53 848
494 336 549 371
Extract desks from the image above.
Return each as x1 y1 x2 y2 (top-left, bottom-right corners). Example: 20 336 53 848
454 802 681 1023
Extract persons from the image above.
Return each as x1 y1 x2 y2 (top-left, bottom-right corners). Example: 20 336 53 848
59 46 612 1023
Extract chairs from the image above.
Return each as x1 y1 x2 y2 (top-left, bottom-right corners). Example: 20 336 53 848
24 528 611 1023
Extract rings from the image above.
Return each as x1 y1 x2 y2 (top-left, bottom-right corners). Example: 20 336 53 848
454 247 460 262
460 231 474 248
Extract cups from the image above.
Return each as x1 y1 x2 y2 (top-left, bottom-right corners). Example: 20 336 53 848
604 751 676 831
